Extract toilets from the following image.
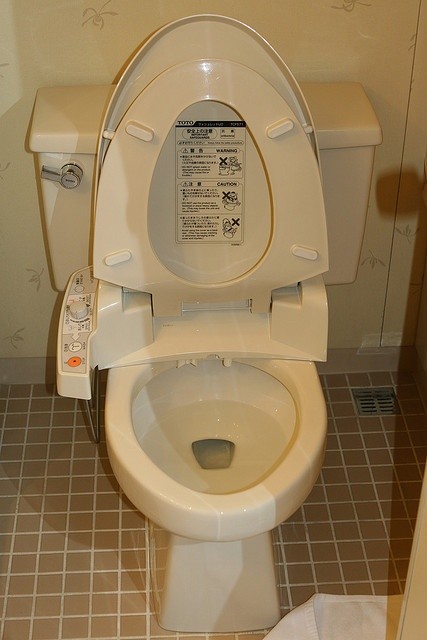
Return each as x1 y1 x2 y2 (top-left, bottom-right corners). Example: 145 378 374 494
26 13 385 631
